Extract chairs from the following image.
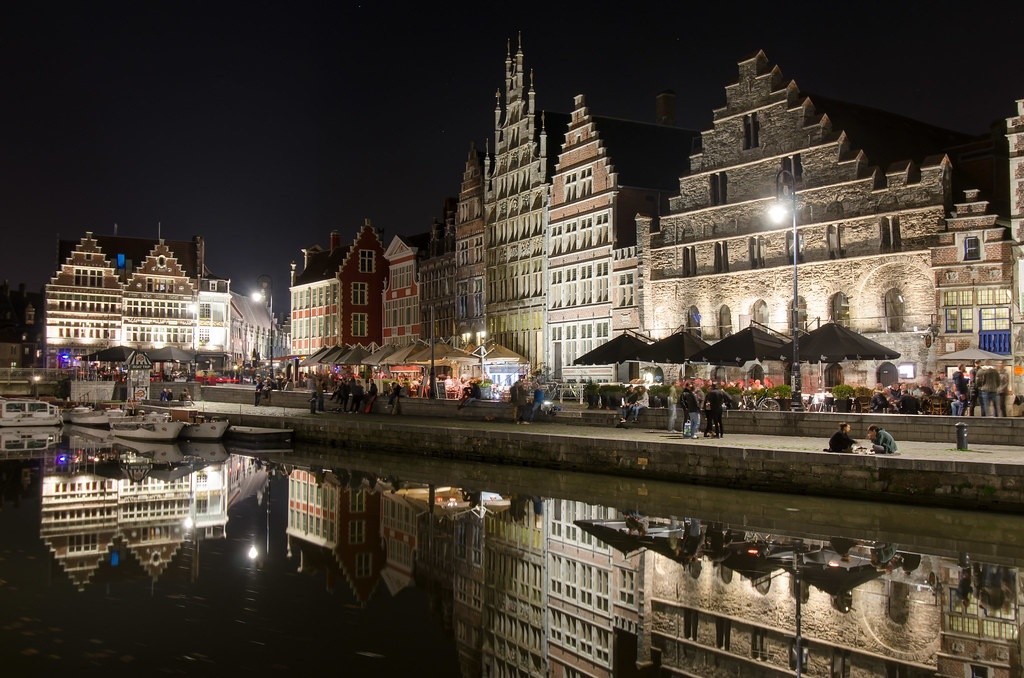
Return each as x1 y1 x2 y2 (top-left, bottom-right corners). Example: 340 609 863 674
929 395 953 415
900 395 919 415
859 400 871 413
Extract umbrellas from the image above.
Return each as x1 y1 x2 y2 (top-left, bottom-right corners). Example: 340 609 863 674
76 463 206 481
299 335 527 386
936 347 1013 369
574 520 898 598
382 487 511 521
82 345 193 363
568 317 901 392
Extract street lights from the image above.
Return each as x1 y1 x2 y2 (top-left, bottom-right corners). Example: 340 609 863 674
770 171 803 412
252 275 274 388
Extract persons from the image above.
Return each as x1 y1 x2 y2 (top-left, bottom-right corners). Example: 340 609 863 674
406 375 481 410
828 538 1015 620
509 380 544 424
828 359 1010 453
622 513 735 567
89 365 190 401
620 382 732 440
457 488 546 522
331 467 404 494
231 364 402 414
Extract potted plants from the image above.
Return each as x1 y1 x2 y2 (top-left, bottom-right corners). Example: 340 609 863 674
382 378 391 394
657 386 671 408
853 386 875 413
402 380 411 398
194 370 205 385
608 384 625 410
724 384 794 411
647 385 660 407
206 369 216 386
831 384 854 413
470 379 493 400
583 384 601 410
510 380 527 403
599 385 611 410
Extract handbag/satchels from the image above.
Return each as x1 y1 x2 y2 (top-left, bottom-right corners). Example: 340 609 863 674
684 420 691 437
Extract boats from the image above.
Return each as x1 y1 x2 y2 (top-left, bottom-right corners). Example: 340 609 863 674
181 416 229 438
228 426 293 442
109 412 190 439
61 406 90 422
0 426 230 467
68 408 128 425
225 442 294 454
1 396 62 426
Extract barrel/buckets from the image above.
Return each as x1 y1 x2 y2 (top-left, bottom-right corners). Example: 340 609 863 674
683 420 691 436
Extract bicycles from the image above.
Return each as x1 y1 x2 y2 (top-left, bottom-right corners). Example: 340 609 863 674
740 392 780 411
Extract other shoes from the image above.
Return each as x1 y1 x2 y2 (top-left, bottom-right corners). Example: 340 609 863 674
691 435 698 439
704 435 708 437
714 435 719 438
721 435 723 437
669 429 677 434
620 418 626 423
632 417 639 423
